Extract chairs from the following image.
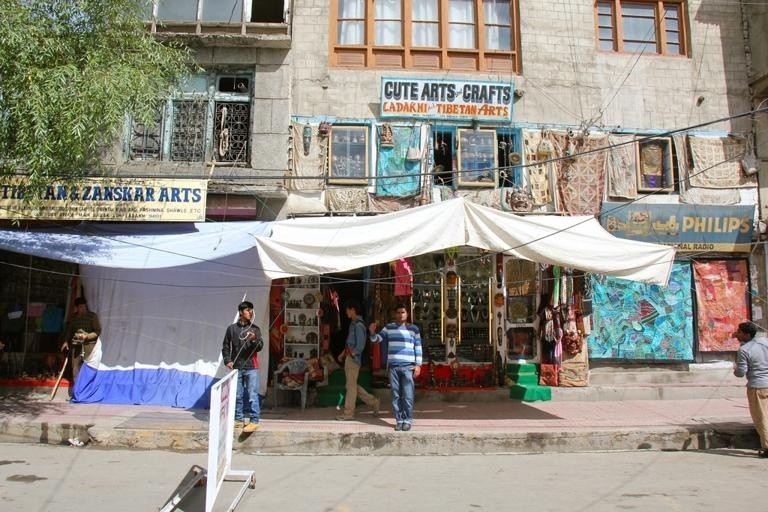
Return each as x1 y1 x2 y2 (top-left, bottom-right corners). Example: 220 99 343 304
273 359 316 411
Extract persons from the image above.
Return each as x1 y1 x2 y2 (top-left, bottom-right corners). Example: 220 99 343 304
60 297 101 383
336 298 381 421
734 322 768 458
222 301 263 432
368 304 423 431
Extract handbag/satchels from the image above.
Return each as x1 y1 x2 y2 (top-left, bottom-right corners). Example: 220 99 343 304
539 365 558 386
363 329 370 359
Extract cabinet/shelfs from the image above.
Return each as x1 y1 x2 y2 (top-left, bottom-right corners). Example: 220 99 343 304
284 276 320 358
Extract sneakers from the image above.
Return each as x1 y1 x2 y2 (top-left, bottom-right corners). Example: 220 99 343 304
395 422 411 431
373 394 383 417
234 418 246 428
241 421 259 433
334 412 356 421
